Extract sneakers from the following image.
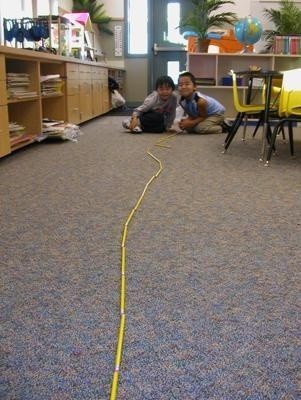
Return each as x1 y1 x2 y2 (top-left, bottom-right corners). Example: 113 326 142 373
122 117 143 133
218 119 234 133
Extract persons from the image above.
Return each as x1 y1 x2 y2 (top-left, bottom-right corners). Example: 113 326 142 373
121 75 178 133
178 72 227 135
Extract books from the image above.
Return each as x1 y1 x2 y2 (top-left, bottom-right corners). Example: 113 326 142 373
6 72 38 100
9 121 31 147
40 74 64 96
42 117 73 139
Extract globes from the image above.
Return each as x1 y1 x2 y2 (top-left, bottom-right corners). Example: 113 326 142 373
234 15 262 54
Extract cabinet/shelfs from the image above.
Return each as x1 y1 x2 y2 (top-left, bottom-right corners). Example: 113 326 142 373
185 52 301 90
0 55 66 153
66 63 127 126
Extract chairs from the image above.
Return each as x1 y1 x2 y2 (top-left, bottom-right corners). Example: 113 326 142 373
221 68 301 167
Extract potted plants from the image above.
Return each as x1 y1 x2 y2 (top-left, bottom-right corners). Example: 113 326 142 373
175 1 240 52
